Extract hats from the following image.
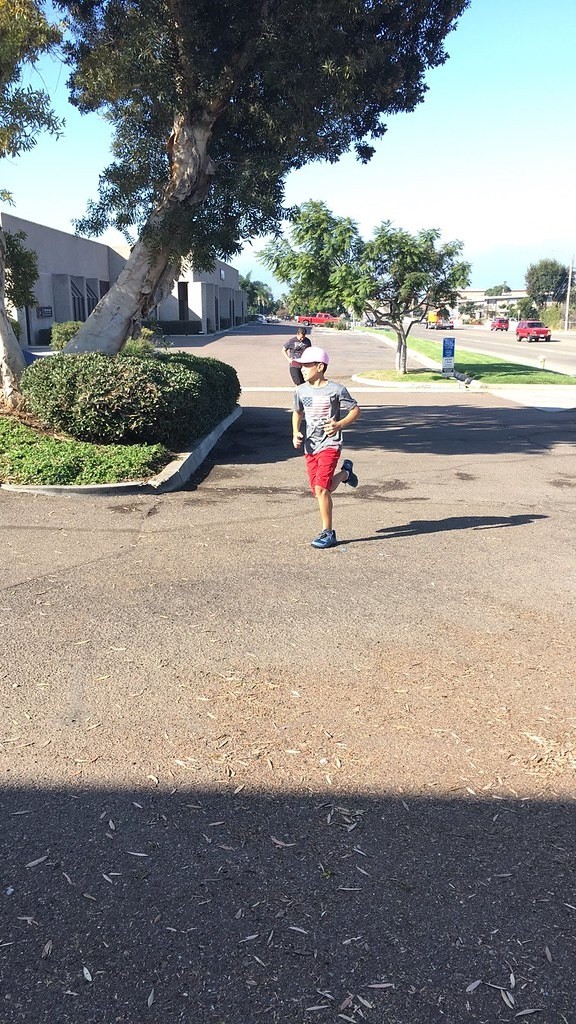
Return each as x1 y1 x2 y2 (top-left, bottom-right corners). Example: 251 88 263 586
292 346 329 366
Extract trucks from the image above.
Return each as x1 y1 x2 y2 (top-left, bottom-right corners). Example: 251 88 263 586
425 311 454 330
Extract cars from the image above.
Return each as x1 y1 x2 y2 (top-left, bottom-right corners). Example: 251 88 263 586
285 315 292 321
419 318 426 325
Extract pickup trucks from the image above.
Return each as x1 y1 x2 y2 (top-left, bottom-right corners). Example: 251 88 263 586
298 313 339 327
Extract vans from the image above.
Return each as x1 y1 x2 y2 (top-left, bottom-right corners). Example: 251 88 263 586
253 315 267 323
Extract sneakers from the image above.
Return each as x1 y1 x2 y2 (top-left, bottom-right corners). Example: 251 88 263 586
340 459 358 488
310 529 338 549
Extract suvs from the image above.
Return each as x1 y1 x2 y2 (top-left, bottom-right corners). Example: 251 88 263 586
491 319 509 332
515 321 552 343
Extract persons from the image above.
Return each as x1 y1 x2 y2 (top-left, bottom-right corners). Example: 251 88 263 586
281 327 311 421
292 347 362 548
